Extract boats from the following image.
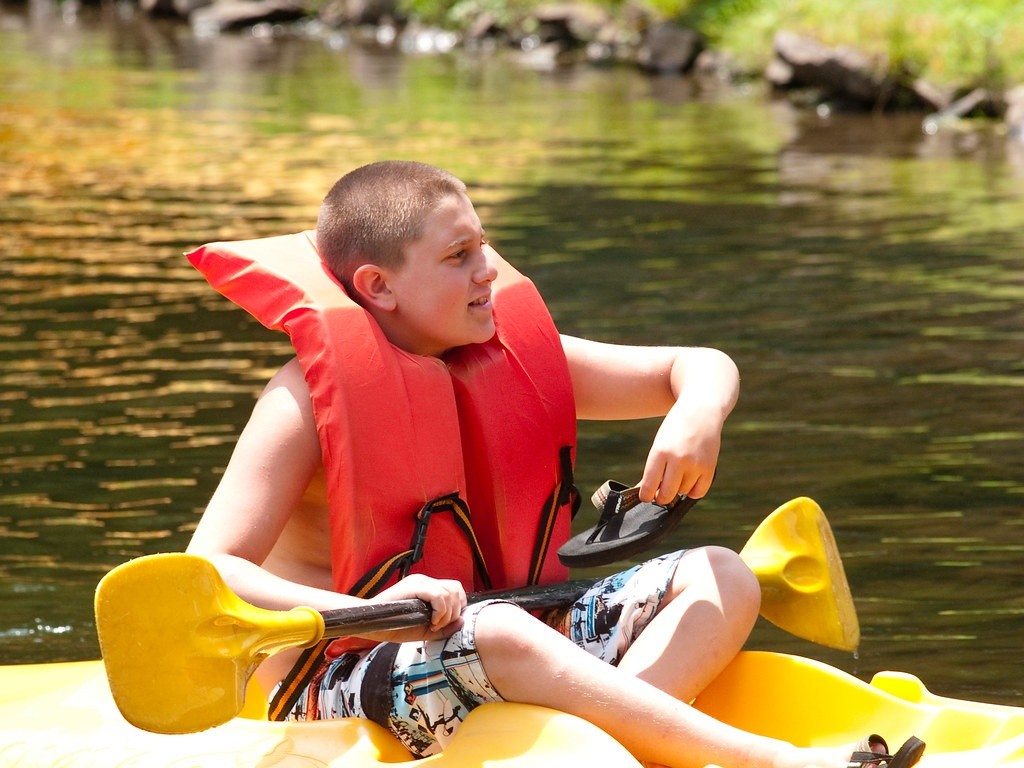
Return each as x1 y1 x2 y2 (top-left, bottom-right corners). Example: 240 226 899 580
0 645 1024 768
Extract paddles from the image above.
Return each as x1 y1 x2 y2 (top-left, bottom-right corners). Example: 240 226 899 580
89 492 864 736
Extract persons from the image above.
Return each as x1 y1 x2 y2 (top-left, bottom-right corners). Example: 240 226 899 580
183 160 928 768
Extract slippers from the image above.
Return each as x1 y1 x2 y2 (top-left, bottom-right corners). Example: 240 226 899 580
847 734 926 768
556 468 717 567
